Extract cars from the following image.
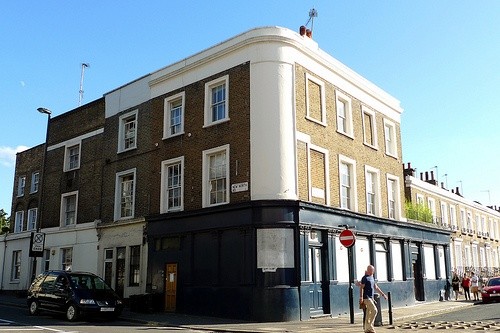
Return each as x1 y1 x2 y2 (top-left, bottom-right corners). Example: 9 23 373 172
480 277 500 303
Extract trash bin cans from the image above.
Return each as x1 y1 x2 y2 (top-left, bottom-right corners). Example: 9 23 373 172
362 293 384 328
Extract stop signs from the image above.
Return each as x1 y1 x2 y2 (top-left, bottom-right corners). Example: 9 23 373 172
339 229 356 248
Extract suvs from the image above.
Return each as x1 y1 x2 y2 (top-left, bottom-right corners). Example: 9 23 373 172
26 270 124 323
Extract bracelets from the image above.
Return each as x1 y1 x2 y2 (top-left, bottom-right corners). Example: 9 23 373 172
381 293 386 296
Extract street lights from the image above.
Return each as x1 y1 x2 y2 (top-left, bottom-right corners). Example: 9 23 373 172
25 107 51 292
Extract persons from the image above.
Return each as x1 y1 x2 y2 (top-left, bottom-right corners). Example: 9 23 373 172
355 265 389 333
452 272 461 301
461 273 471 302
470 271 480 301
